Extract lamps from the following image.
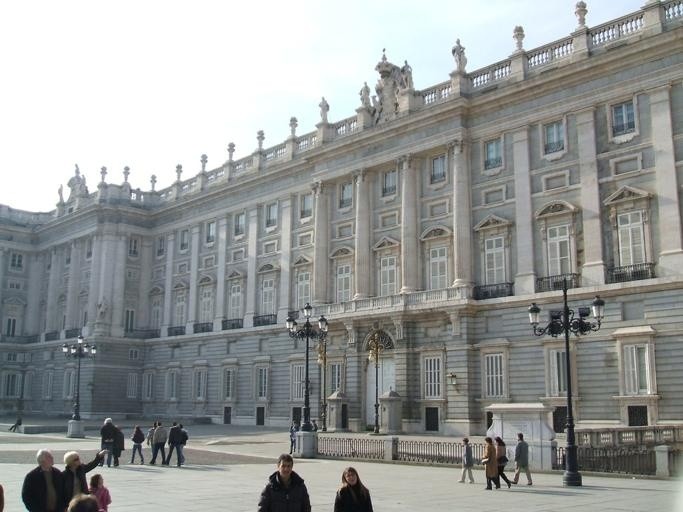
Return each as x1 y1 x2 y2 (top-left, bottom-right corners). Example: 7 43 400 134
445 372 458 387
87 382 95 391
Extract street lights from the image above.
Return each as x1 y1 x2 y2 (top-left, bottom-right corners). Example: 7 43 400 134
365 333 388 432
528 277 604 486
285 302 328 432
60 335 96 420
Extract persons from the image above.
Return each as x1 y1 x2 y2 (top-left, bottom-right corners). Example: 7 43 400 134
310 419 318 431
97 296 109 319
452 38 465 71
457 433 532 490
21 449 67 512
401 60 412 88
334 467 373 512
8 415 22 432
0 484 6 512
67 493 99 512
89 474 112 512
257 454 311 512
289 421 299 454
319 97 329 121
100 417 188 467
359 82 370 107
60 449 110 509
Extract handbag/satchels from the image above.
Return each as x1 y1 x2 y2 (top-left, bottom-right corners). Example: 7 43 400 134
481 457 490 464
499 456 508 464
